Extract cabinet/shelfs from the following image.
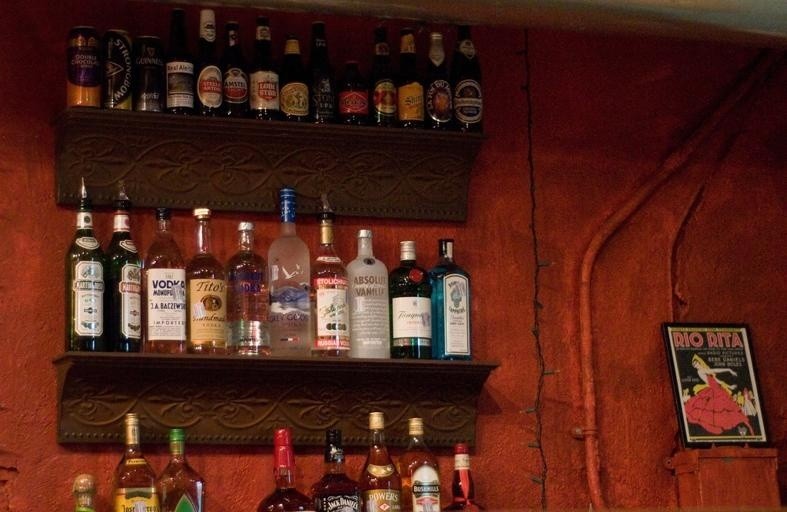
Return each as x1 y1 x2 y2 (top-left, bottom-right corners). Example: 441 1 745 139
663 449 786 512
49 108 503 449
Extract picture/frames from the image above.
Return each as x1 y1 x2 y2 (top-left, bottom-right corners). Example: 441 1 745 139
661 320 771 448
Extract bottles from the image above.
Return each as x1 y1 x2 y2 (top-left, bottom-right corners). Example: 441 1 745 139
278 32 309 121
268 187 311 359
338 59 368 125
307 19 335 124
249 17 280 120
195 8 222 116
225 220 272 356
346 229 390 360
310 211 351 360
367 25 398 127
103 180 142 352
156 428 204 512
142 207 187 353
396 26 425 128
163 6 193 113
425 33 452 132
66 177 105 352
110 413 160 512
222 20 249 118
185 206 227 355
257 412 485 512
388 240 432 360
428 238 471 361
451 23 484 133
